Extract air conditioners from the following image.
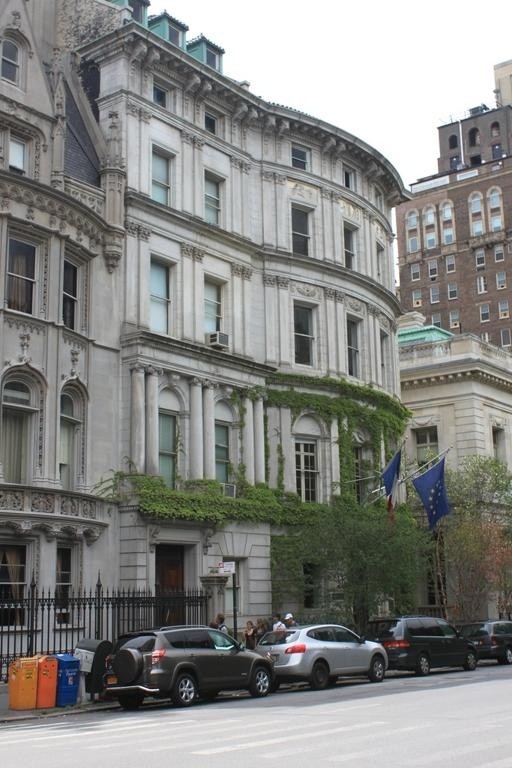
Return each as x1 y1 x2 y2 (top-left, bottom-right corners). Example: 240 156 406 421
206 330 229 350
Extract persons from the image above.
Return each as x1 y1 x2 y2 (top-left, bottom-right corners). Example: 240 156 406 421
209 611 300 649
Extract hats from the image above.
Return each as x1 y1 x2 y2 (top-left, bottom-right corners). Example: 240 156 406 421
284 613 293 620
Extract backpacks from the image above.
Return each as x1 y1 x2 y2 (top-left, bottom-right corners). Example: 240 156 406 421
220 624 234 638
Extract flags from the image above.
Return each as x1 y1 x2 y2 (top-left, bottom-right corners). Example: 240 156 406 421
379 451 403 524
410 455 450 533
